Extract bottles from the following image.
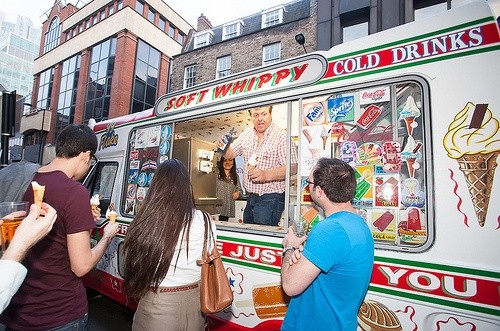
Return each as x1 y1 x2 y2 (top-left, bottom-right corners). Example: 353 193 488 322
217 128 234 156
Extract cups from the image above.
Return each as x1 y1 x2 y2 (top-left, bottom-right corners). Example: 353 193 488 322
0 201 29 255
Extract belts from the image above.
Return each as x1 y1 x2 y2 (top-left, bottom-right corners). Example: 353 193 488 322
148 284 198 292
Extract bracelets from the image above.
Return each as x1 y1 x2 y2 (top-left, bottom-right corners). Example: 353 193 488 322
283 246 295 256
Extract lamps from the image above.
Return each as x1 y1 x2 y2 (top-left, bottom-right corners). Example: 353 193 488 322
295 32 307 54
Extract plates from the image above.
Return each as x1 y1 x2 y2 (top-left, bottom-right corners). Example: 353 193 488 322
356 142 383 166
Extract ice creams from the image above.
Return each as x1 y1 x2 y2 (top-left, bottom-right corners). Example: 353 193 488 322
400 96 421 136
32 181 45 218
400 135 422 177
107 210 118 225
248 157 257 182
321 127 331 150
90 194 100 211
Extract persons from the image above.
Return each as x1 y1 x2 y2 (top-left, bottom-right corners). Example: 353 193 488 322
0 202 57 315
216 156 243 222
224 105 298 226
4 124 121 331
122 158 217 331
280 157 374 331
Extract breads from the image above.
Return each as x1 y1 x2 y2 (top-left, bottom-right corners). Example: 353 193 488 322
252 286 290 319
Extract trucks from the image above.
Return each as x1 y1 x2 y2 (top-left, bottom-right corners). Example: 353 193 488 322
58 0 500 331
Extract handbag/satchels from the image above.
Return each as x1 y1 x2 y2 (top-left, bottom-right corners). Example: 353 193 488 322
196 211 233 315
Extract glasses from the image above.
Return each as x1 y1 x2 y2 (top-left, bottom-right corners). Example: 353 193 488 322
305 177 320 186
91 152 98 167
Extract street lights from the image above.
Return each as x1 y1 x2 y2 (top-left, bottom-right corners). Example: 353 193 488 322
22 102 46 165
